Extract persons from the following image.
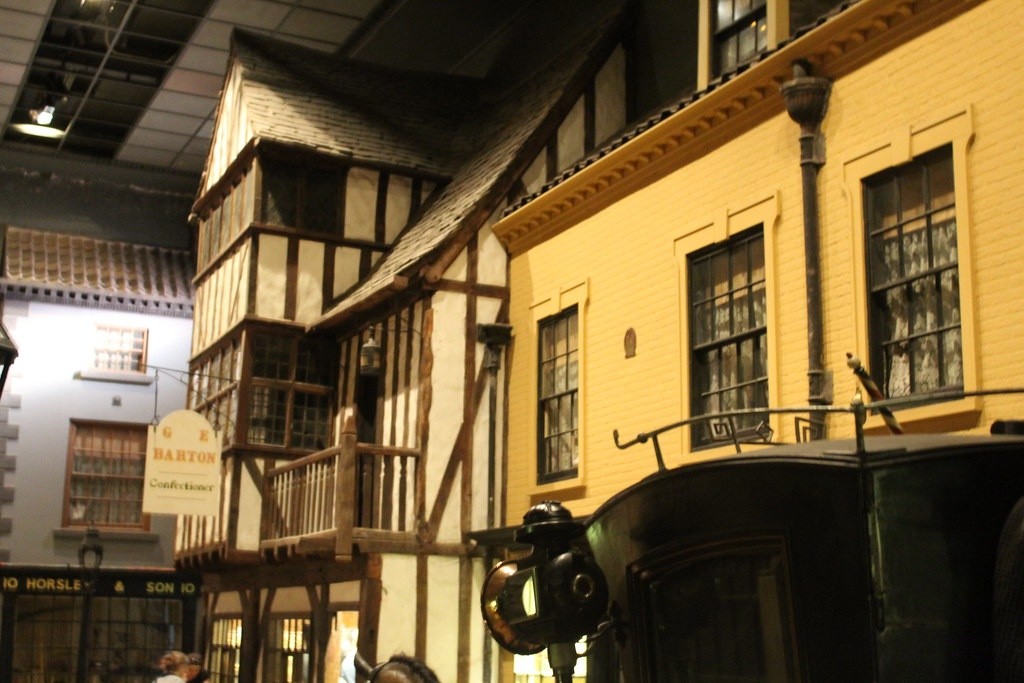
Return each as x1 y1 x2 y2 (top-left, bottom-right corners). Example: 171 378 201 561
152 651 211 683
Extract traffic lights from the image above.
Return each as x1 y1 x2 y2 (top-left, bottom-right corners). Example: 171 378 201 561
503 566 553 629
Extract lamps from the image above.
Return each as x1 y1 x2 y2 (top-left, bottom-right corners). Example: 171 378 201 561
359 325 383 377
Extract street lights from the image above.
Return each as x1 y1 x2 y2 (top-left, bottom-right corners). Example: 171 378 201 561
73 520 104 683
